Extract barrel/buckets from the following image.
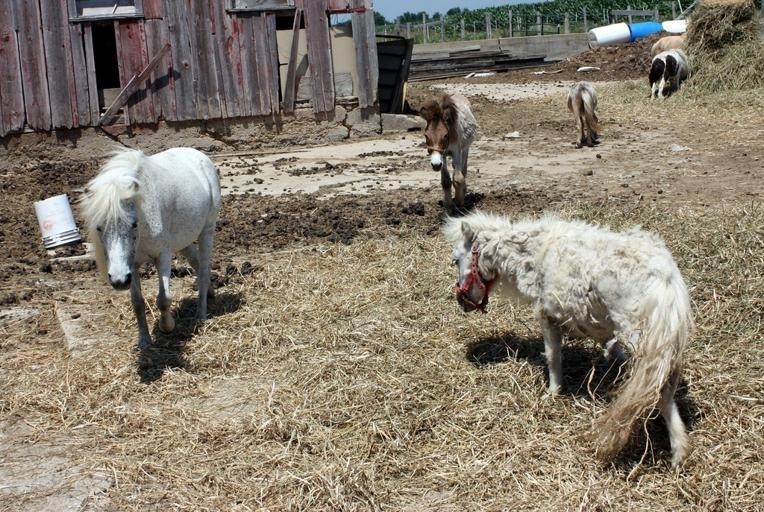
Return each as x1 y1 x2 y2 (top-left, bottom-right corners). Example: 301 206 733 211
663 19 687 33
33 193 80 251
587 22 631 51
628 22 663 36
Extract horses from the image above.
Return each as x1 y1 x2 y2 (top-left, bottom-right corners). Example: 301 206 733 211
440 210 694 470
650 32 688 100
68 145 222 373
417 91 478 209
566 80 598 149
